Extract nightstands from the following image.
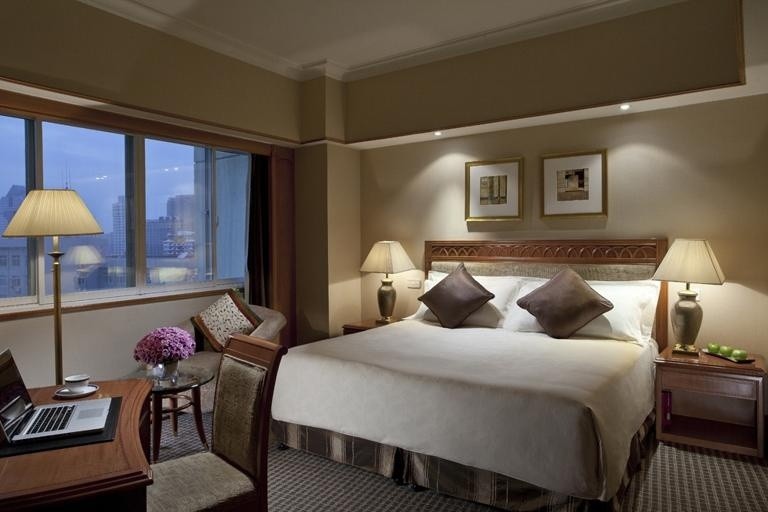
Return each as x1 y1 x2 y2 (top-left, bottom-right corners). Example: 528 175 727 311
341 321 381 337
651 342 768 465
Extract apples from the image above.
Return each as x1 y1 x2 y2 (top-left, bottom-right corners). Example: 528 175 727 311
732 349 747 360
720 345 733 357
707 342 720 354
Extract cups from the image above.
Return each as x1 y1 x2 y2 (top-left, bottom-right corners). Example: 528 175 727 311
145 363 165 391
64 375 90 393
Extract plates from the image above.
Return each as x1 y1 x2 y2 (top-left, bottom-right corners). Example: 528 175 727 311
53 384 100 398
702 348 756 363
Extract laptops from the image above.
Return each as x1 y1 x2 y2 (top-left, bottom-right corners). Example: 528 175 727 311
1 348 112 444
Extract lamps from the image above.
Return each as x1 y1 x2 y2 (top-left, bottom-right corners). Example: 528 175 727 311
358 240 418 325
651 237 726 355
4 188 106 387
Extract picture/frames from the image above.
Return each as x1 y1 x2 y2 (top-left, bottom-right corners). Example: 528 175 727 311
539 150 609 220
464 156 526 223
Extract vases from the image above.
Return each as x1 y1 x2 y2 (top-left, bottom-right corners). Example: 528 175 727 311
163 361 179 377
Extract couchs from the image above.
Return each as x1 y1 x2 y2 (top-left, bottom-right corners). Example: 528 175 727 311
174 302 288 413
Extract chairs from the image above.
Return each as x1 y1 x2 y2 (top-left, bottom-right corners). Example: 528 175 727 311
144 331 288 512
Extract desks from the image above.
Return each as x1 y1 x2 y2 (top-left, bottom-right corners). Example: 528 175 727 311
0 378 156 512
129 365 215 454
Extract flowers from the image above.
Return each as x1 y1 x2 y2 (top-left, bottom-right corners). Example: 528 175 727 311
132 326 197 362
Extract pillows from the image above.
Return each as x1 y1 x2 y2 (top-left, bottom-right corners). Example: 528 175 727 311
417 262 496 328
415 279 521 328
190 288 264 354
424 271 453 277
496 276 662 348
516 267 614 339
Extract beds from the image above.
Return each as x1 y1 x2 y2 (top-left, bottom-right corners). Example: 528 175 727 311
270 239 669 512
